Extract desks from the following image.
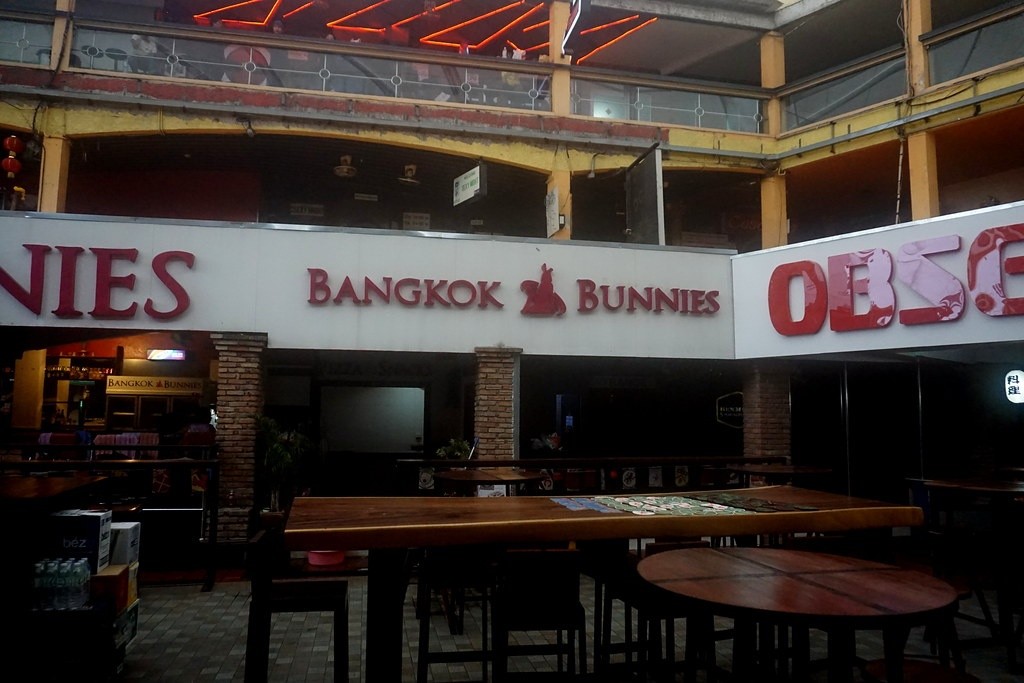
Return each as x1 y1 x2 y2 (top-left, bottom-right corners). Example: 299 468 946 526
723 465 833 487
284 496 924 683
636 546 956 683
922 481 1024 551
433 469 542 497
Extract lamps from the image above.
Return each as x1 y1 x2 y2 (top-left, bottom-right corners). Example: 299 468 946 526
759 159 781 176
236 116 256 137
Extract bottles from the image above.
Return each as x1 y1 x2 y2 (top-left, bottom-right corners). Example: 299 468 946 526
21 365 215 461
32 556 90 612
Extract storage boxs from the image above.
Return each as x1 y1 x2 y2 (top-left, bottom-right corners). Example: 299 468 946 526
48 509 141 614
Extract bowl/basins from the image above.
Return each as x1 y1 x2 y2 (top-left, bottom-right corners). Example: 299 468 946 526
308 550 346 565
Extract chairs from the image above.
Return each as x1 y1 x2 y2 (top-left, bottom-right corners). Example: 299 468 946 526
242 518 1001 683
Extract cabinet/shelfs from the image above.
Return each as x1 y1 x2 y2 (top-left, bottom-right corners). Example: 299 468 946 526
10 346 123 431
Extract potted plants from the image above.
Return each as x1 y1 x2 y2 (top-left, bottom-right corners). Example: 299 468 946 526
436 438 471 471
